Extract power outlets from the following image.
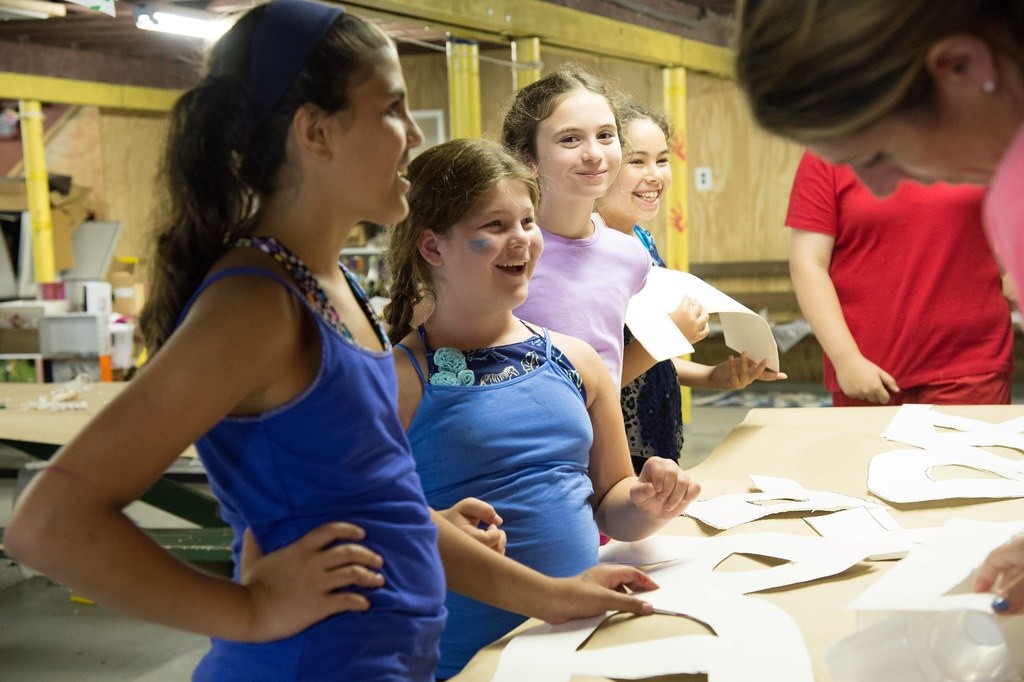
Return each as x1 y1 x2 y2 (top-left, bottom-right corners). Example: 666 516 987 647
696 167 710 187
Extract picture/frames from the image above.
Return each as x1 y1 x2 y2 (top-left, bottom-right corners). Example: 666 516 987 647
406 110 441 160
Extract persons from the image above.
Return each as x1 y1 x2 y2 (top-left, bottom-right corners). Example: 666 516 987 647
370 134 703 681
733 0 1022 621
786 131 1017 408
2 0 658 681
583 100 791 488
495 66 653 556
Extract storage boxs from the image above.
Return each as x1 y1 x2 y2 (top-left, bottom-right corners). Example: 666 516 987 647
0 273 146 370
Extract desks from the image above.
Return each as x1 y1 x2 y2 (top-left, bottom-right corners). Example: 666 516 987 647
444 406 1024 681
0 380 237 588
